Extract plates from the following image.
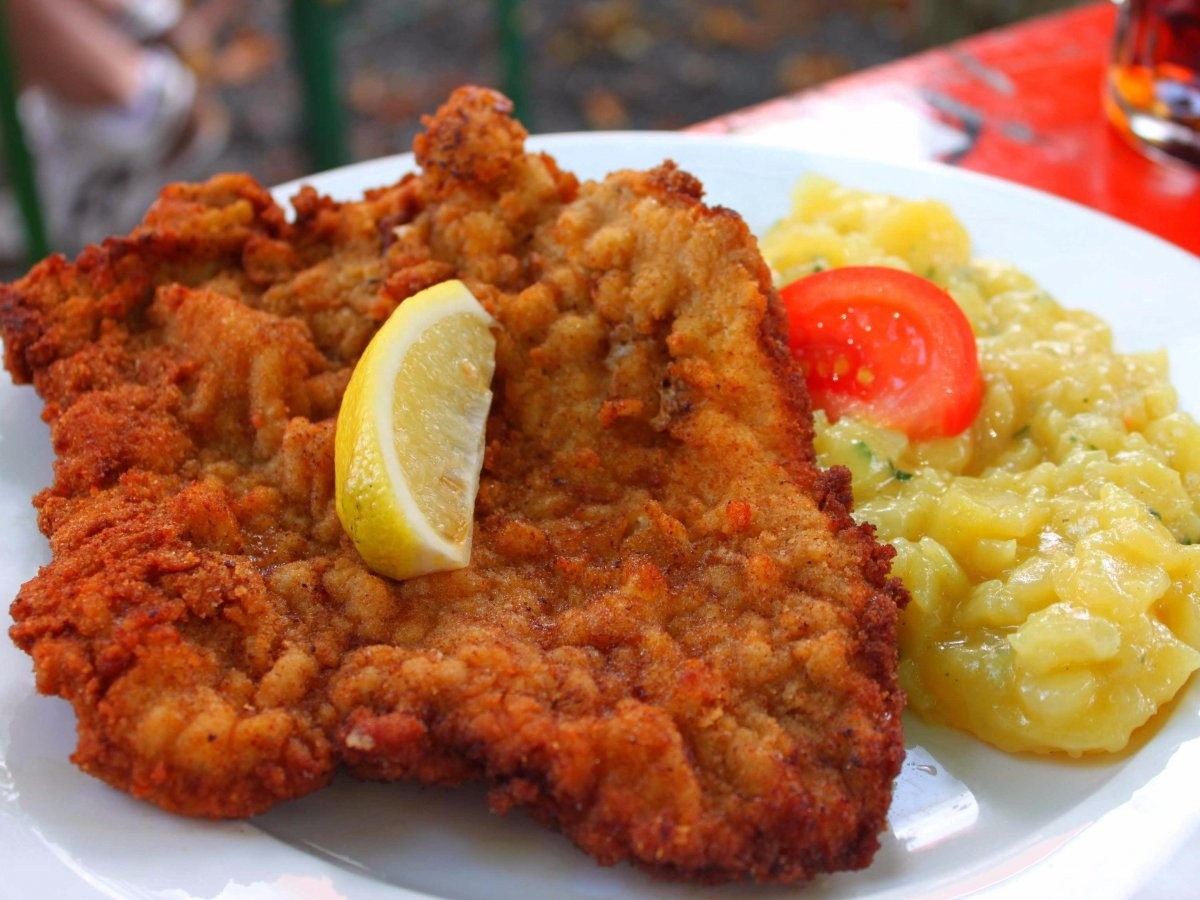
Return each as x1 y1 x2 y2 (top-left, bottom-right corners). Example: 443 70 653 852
0 131 1200 896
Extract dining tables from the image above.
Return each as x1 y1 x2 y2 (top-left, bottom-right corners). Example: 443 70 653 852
685 0 1200 252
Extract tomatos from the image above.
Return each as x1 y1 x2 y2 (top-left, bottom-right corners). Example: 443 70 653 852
772 266 982 447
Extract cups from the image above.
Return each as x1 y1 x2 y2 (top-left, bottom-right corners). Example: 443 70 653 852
1101 0 1200 173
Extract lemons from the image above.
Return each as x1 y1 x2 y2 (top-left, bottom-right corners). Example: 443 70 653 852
332 279 496 569
